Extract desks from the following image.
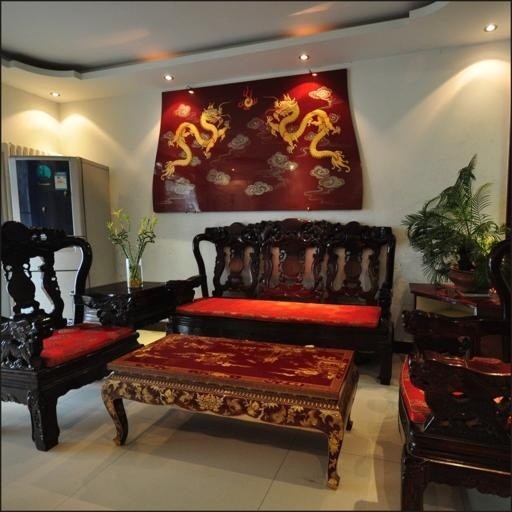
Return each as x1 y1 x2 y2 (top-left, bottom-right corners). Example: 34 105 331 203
101 333 359 489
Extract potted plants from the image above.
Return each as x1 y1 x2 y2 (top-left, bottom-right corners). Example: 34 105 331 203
103 207 159 288
396 152 512 298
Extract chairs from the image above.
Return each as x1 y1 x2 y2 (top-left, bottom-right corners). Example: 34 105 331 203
399 237 512 512
0 220 145 451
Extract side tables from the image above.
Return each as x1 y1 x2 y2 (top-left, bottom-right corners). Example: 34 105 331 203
407 281 503 360
65 281 169 334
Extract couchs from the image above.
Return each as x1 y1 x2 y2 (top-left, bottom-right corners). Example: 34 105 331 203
164 217 396 384
